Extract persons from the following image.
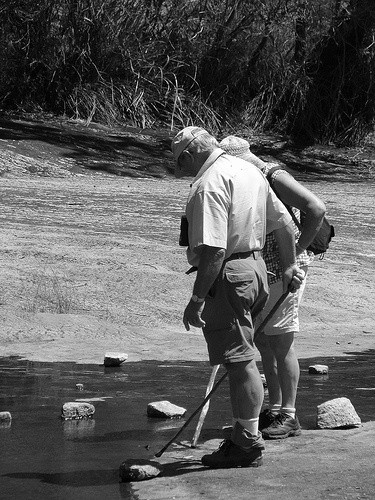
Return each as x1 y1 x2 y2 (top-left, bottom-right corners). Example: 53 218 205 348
217 135 327 439
170 127 306 467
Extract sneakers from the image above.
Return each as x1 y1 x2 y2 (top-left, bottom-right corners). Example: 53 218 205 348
258 410 278 432
202 440 264 467
260 413 301 439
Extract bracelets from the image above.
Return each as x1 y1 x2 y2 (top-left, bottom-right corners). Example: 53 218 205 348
296 242 306 252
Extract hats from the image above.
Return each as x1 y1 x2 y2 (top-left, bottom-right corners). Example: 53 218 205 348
170 126 210 179
219 135 267 171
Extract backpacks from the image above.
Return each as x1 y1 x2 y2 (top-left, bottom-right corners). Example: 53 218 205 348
266 166 336 255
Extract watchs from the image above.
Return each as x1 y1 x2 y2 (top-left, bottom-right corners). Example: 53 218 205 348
191 294 205 303
